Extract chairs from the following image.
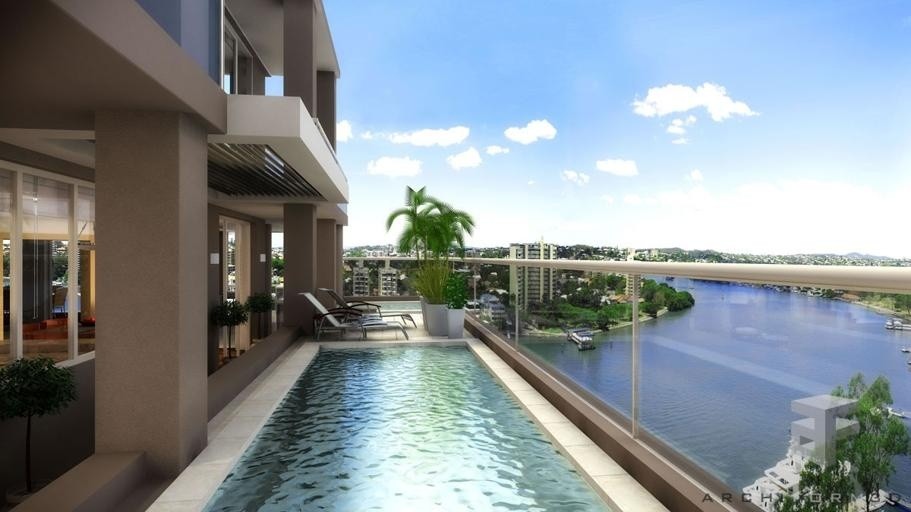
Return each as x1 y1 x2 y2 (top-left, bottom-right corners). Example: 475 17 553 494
297 284 417 342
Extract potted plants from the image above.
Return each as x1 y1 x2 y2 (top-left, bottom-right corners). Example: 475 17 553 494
390 185 476 339
0 354 80 512
207 291 275 363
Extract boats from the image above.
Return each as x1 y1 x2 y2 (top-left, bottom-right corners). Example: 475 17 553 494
567 328 594 350
885 318 911 330
